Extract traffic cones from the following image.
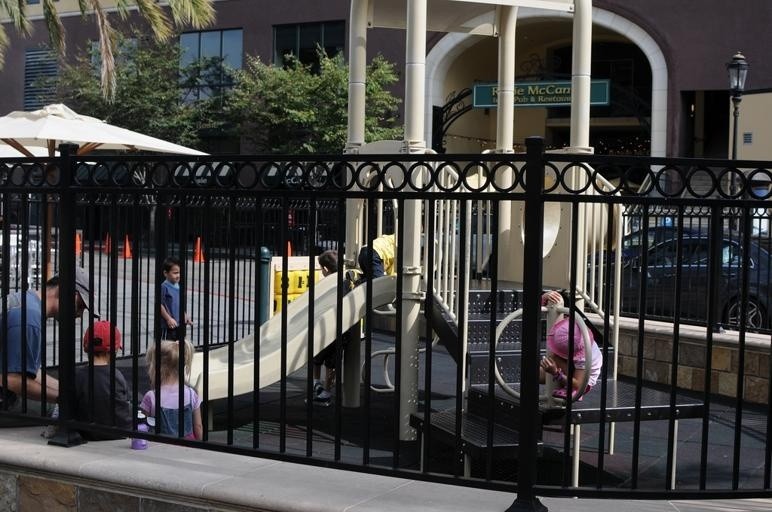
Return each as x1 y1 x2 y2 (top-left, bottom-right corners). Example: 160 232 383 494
193 237 204 262
76 233 81 256
103 233 112 254
120 234 132 258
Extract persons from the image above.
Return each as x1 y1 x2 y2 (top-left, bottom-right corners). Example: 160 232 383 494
344 233 397 295
313 250 338 405
0 256 205 443
539 290 604 401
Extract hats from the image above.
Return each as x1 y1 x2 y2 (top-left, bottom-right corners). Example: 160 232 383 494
84 320 122 353
54 266 101 318
546 316 594 361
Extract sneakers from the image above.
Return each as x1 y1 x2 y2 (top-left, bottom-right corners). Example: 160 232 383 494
344 270 358 293
304 382 332 407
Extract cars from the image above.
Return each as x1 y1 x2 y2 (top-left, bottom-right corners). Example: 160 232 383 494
585 225 772 333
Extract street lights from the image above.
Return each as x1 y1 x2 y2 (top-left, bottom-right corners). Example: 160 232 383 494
724 50 749 231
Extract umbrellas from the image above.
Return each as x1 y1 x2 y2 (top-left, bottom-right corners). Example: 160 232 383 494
0 102 211 282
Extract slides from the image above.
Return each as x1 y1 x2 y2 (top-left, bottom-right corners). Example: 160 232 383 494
190 269 398 402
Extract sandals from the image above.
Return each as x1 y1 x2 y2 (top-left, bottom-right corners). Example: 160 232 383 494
552 386 584 402
